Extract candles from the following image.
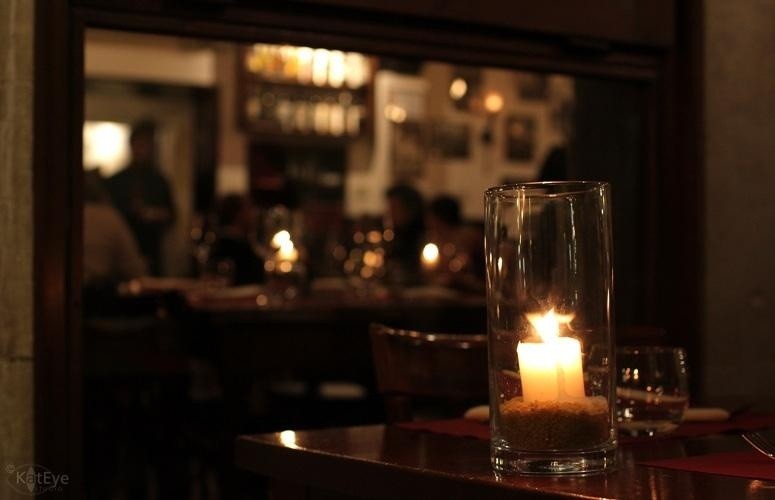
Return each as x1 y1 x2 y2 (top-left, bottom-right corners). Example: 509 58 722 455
517 310 585 402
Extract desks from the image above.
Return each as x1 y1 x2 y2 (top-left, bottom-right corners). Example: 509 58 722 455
237 410 773 500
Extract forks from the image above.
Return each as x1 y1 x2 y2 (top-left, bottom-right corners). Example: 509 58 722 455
739 432 775 461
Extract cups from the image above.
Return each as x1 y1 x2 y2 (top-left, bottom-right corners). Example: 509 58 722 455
484 181 612 475
614 347 688 436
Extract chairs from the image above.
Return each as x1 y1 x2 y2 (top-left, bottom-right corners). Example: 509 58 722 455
369 317 665 422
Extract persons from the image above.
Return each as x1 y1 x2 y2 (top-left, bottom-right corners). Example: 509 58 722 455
78 120 484 318
